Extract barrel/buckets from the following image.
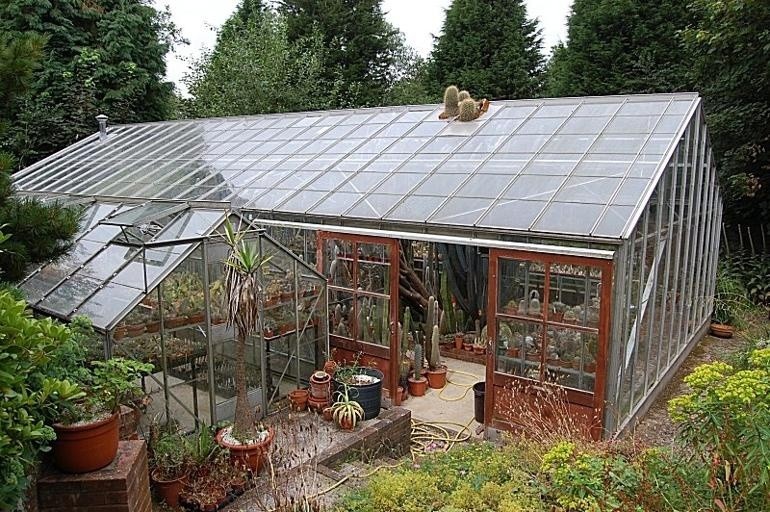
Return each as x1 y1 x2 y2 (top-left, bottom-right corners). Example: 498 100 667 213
332 367 384 419
473 381 486 422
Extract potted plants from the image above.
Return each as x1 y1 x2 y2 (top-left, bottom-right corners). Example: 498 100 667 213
710 269 751 339
0 243 603 512
